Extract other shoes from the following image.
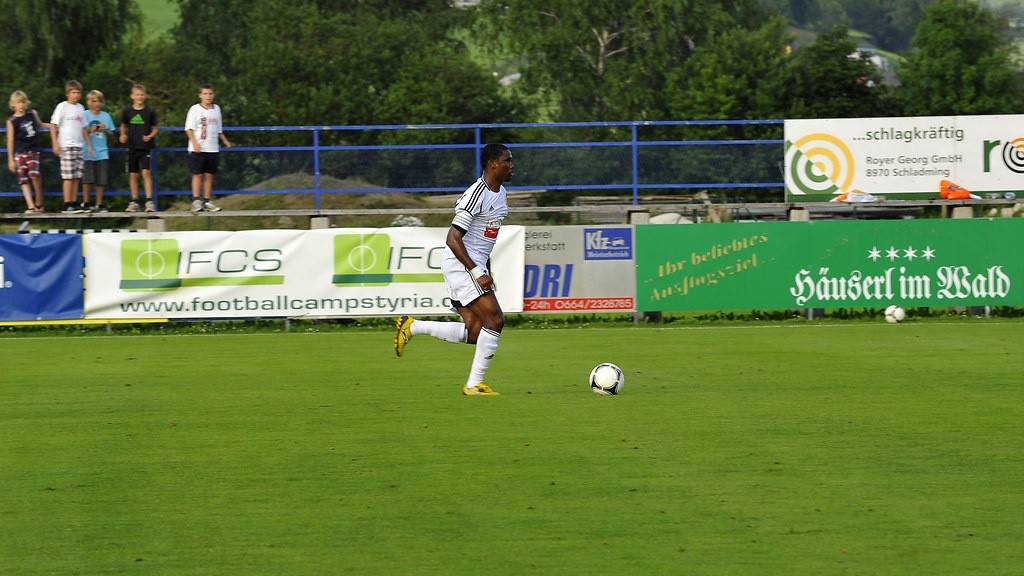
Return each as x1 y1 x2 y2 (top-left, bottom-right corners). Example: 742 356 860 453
25 207 35 215
94 204 108 212
73 201 91 212
60 202 84 214
81 203 95 210
34 205 44 213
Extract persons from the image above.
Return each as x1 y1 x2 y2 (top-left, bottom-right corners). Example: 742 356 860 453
184 83 232 212
119 84 159 213
78 90 116 213
393 144 515 397
6 90 58 215
50 80 93 214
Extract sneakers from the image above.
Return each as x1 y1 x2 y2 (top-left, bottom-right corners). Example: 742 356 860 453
463 382 501 396
191 200 204 212
394 316 414 357
145 201 155 212
203 201 222 212
125 202 141 212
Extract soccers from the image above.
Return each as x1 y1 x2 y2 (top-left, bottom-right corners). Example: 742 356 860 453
884 305 906 324
589 362 626 396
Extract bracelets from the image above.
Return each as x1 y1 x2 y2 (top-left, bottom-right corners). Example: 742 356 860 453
470 266 485 279
